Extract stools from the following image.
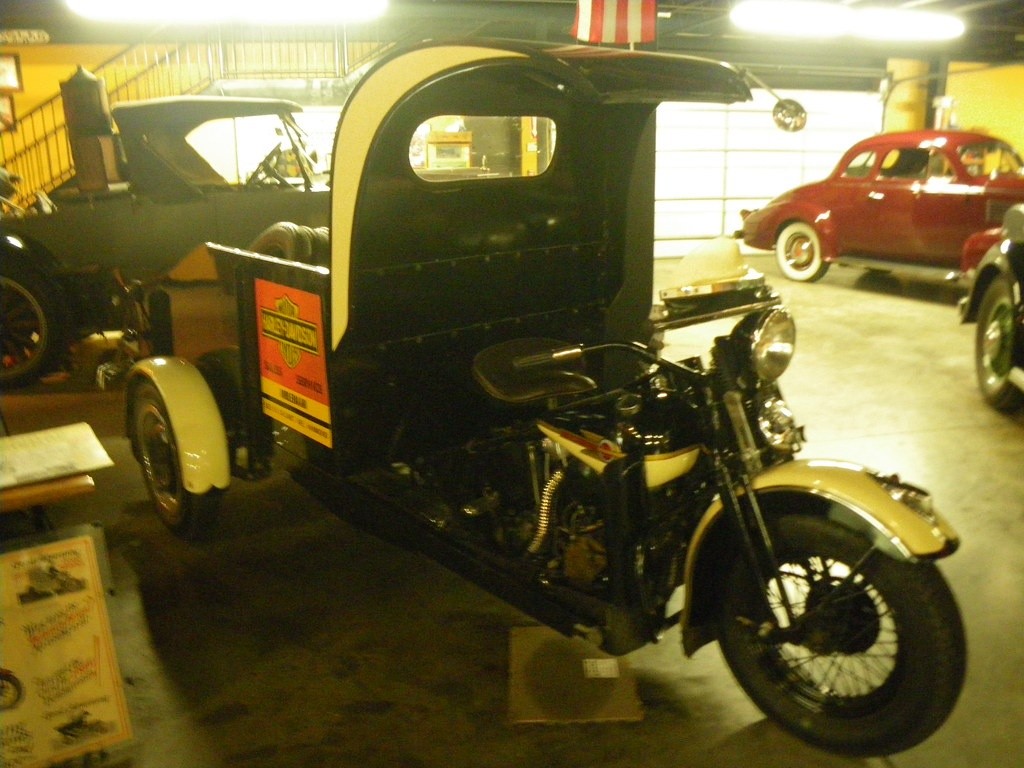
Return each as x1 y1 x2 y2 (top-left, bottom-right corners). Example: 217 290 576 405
472 336 600 414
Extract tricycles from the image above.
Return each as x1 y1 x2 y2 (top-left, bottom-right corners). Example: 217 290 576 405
122 22 974 755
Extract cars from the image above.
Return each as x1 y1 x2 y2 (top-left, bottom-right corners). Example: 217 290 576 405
738 122 1022 283
963 201 1024 413
1 86 329 399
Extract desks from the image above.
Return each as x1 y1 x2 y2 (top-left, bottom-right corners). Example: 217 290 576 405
0 474 96 530
